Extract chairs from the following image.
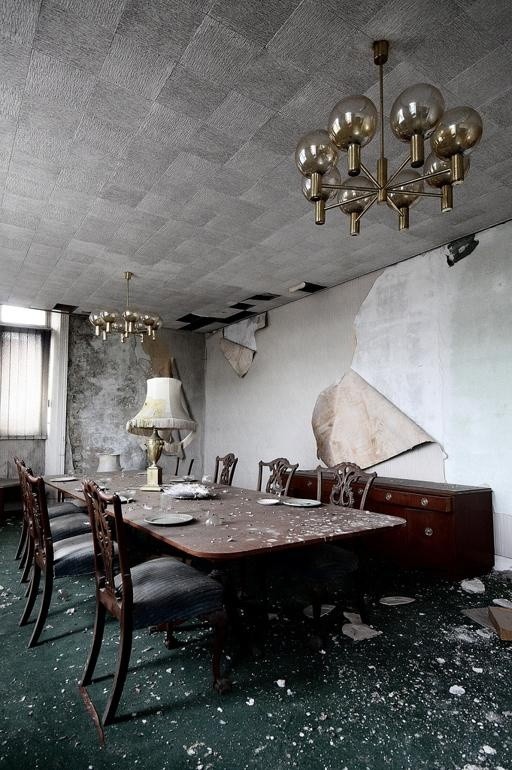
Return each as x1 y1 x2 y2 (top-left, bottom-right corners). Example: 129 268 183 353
81 479 241 724
19 470 120 648
267 464 376 637
12 454 299 595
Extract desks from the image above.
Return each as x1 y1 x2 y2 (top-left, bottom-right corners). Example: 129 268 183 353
0 478 21 529
37 471 408 694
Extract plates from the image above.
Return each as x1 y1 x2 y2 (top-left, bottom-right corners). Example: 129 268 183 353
283 497 323 509
302 604 336 620
50 476 76 483
379 595 414 608
256 498 280 506
143 512 194 526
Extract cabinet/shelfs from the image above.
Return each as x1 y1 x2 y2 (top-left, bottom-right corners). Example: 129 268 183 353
281 471 494 582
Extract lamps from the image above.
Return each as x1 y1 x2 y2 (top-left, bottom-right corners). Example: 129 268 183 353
89 271 164 343
126 376 197 492
295 40 481 236
